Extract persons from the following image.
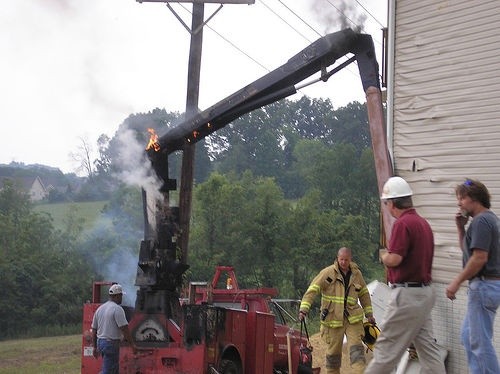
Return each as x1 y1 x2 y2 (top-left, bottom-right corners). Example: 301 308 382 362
299 247 376 374
445 180 499 374
361 177 445 374
92 286 138 374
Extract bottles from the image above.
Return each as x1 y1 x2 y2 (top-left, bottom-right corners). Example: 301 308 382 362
226 277 233 290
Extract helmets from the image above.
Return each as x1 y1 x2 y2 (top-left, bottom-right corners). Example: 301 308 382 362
380 177 413 200
109 284 127 295
360 322 382 353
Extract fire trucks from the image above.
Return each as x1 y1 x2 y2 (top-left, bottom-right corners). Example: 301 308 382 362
81 26 398 374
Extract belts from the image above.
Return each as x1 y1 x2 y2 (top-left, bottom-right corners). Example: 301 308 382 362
389 281 431 289
468 274 500 282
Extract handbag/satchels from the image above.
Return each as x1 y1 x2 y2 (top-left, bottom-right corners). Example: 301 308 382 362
298 346 313 373
